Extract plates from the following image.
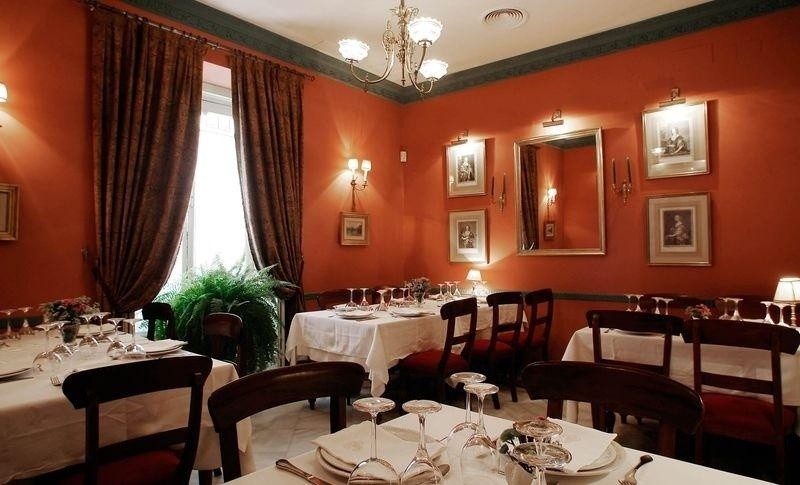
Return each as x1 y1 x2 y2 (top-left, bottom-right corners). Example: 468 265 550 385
389 308 425 317
0 364 31 380
316 447 442 479
145 340 183 354
336 309 374 318
578 444 616 470
496 433 627 476
321 426 446 472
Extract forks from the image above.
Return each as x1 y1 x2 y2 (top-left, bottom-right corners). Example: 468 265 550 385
617 453 654 484
49 376 63 386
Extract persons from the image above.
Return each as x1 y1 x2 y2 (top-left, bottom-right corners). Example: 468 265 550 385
664 124 690 154
665 213 690 245
460 224 476 248
459 156 473 181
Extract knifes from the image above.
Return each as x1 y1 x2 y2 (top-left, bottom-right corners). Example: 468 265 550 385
276 458 332 485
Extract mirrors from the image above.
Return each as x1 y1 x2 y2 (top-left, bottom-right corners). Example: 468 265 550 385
512 125 608 256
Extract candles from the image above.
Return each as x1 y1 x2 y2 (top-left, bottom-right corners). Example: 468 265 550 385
611 158 617 185
626 156 633 185
502 172 506 195
491 176 495 195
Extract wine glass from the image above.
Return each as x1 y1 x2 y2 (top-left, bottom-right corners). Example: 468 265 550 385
347 280 485 309
624 292 673 315
448 372 486 467
444 399 450 485
720 296 790 327
1 303 147 379
517 418 563 458
402 399 446 485
513 442 571 484
461 381 500 478
345 397 400 485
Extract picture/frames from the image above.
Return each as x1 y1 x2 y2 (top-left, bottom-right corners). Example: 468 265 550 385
0 182 22 243
640 99 712 180
445 138 488 199
339 211 371 246
644 190 715 267
448 208 489 264
544 220 555 241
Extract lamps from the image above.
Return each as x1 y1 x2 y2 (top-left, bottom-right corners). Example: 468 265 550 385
0 81 9 127
337 0 450 96
771 276 800 326
467 270 482 296
348 158 372 192
545 185 559 220
